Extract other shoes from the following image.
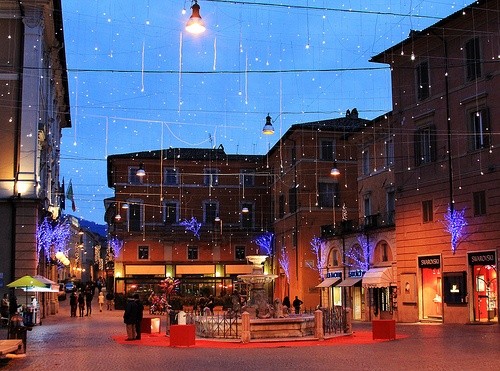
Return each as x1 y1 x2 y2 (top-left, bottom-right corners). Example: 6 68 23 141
136 336 141 340
125 338 136 341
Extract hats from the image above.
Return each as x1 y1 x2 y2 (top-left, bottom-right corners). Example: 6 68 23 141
128 293 134 298
134 294 140 299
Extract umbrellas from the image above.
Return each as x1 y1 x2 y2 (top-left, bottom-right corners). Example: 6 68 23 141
8 275 58 307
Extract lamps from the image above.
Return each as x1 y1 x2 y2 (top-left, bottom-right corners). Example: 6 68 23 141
115 211 121 221
136 162 146 177
241 203 249 213
263 113 275 134
186 0 207 34
331 160 340 175
215 213 221 221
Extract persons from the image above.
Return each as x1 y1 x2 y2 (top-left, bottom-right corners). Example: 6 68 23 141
106 291 113 311
98 292 104 312
282 297 290 309
69 290 92 317
233 289 241 306
198 295 214 314
98 276 105 286
2 293 9 306
293 296 303 314
123 294 143 340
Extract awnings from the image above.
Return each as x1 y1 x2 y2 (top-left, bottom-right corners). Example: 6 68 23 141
362 267 392 289
336 278 362 286
315 278 340 287
